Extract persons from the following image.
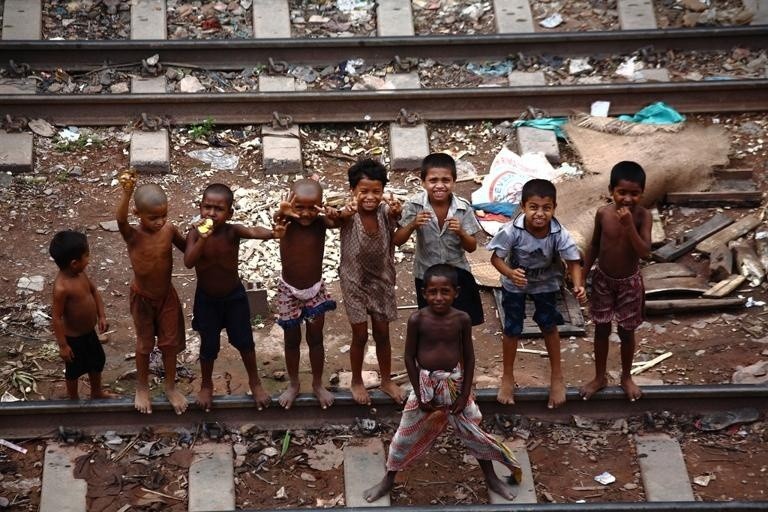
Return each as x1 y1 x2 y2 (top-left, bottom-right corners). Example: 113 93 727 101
362 264 522 503
50 230 120 401
